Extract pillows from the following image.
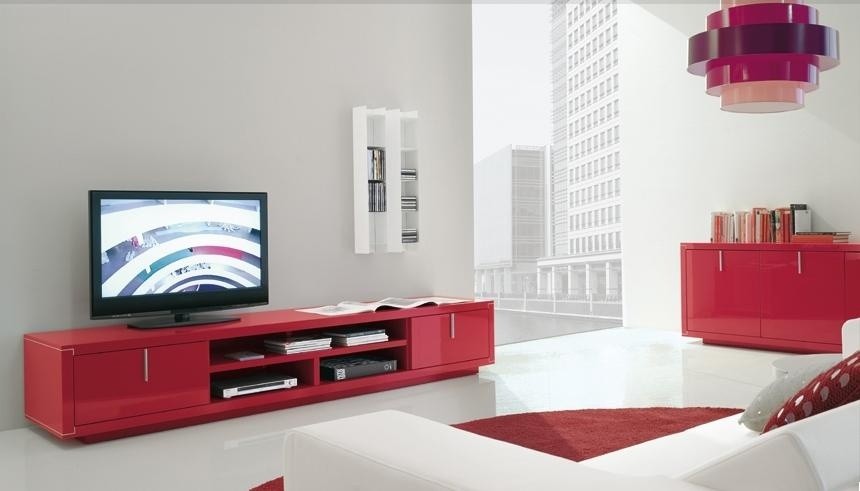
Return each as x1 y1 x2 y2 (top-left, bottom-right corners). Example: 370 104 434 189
738 375 807 431
760 350 860 435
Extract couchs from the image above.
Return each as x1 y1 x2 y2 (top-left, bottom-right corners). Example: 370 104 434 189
283 318 860 491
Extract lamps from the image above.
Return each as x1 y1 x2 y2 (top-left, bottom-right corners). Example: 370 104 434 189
687 0 841 114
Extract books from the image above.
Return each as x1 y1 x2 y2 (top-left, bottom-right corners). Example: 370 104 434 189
293 301 383 317
264 329 390 356
367 148 417 244
375 297 472 309
709 204 852 243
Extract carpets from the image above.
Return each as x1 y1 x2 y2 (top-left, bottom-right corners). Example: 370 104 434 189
250 406 740 491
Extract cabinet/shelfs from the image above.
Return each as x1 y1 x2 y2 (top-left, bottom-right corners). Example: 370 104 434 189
680 242 860 355
352 104 422 254
25 297 497 445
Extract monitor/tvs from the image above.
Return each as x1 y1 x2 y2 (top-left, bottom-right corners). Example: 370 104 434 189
88 190 269 330
